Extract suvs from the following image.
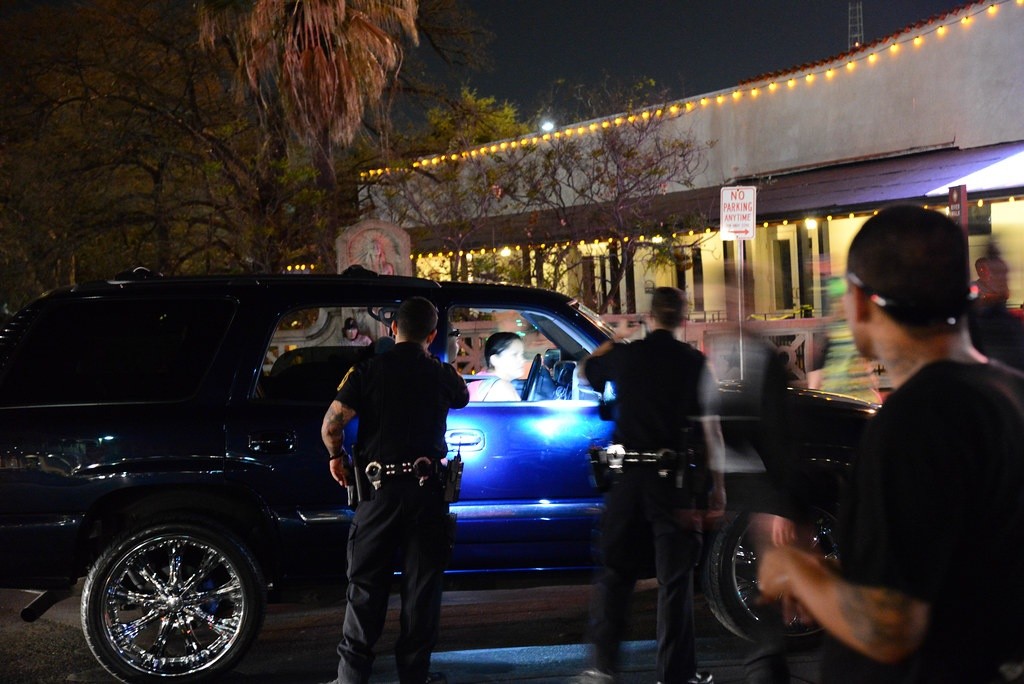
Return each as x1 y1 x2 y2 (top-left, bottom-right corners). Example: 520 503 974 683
0 264 884 683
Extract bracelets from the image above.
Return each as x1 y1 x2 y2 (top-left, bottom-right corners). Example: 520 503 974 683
329 448 345 461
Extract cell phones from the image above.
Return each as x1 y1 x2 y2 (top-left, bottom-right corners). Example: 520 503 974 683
443 460 464 503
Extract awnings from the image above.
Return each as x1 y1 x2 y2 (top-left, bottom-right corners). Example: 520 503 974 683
281 139 1024 275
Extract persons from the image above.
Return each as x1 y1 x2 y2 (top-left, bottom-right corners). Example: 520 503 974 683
321 298 469 683
579 287 729 684
462 330 525 402
448 321 461 363
339 317 373 346
966 256 1023 372
758 202 1024 684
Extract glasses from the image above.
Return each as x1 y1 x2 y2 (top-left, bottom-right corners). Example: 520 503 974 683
846 269 982 327
